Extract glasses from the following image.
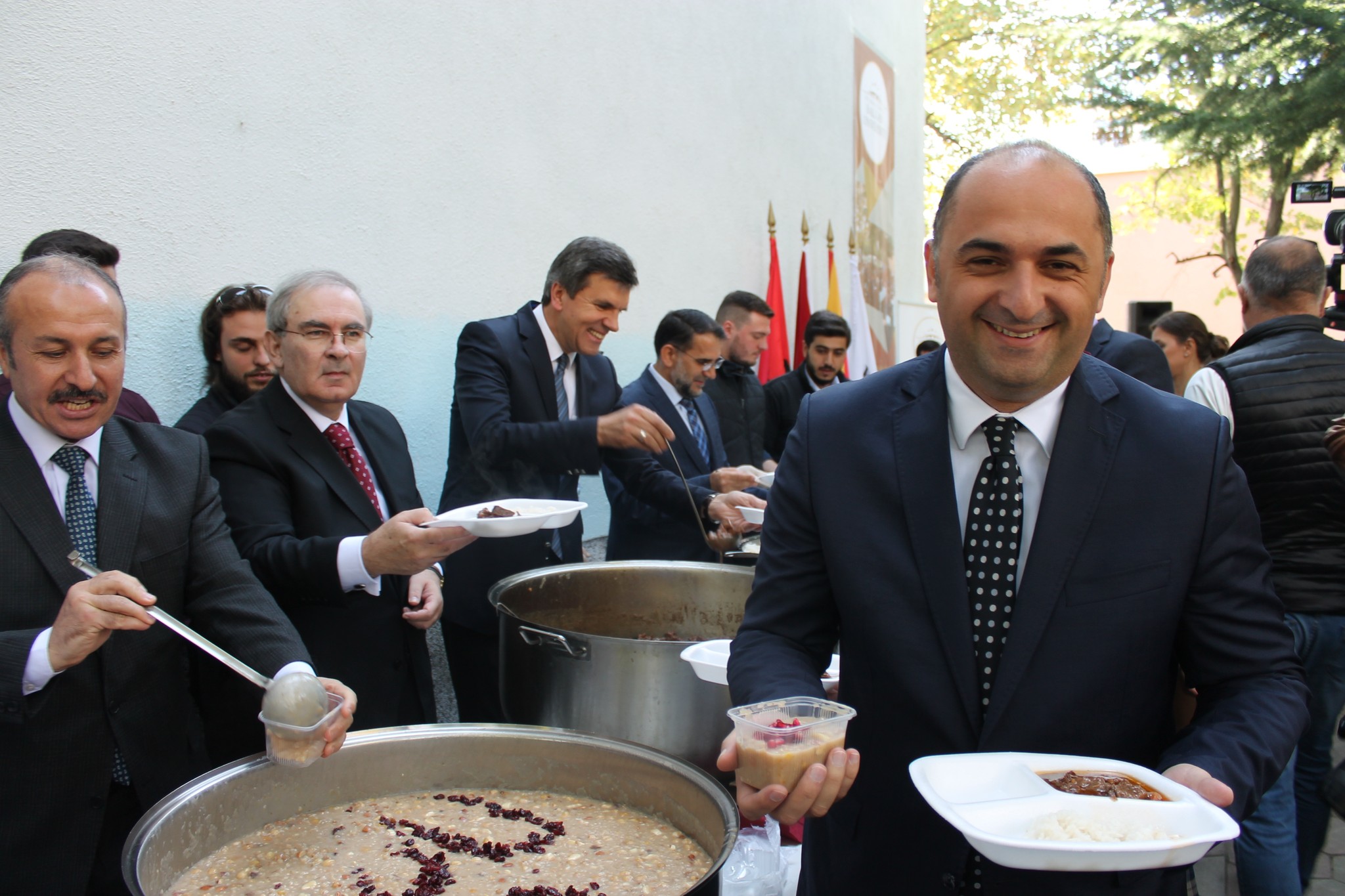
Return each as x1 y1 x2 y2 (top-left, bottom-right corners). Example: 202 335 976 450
275 328 374 353
214 285 273 307
673 346 726 372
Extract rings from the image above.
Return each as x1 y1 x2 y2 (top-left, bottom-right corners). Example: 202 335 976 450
638 429 647 443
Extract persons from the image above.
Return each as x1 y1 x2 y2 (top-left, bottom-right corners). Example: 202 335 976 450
594 308 769 566
12 227 162 437
1082 313 1178 401
202 271 457 725
2 253 357 894
1180 235 1343 896
428 238 678 724
915 339 941 358
761 310 855 463
707 289 780 487
1136 299 1239 447
716 140 1314 896
170 281 291 433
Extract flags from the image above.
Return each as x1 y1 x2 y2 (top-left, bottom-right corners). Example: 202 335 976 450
759 239 792 384
791 251 813 371
822 248 850 384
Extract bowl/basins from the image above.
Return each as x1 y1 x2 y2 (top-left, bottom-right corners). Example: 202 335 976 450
258 691 345 767
725 696 857 794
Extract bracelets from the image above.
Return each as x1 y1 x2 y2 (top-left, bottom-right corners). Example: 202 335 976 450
702 491 722 526
427 562 445 588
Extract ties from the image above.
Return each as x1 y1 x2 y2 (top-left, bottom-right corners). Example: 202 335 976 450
553 354 570 422
49 446 97 580
324 423 385 525
679 397 713 474
959 416 1024 724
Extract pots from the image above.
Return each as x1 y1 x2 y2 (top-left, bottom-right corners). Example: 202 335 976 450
488 560 756 786
120 721 741 896
